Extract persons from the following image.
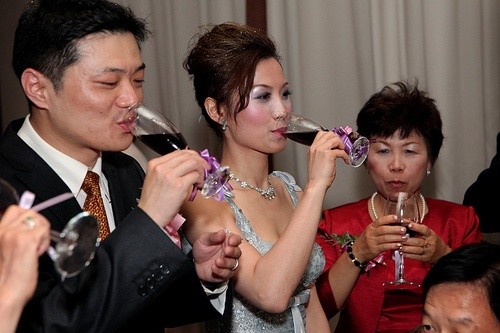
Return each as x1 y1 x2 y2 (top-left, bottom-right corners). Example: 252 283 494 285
0 0 243 332
461 129 500 249
313 77 488 333
180 20 328 333
1 201 51 331
420 236 500 332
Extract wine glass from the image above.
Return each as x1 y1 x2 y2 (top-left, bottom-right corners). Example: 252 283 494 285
122 101 232 198
0 180 99 277
383 192 421 286
276 111 369 168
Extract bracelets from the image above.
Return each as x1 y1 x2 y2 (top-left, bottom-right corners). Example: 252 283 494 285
347 241 369 269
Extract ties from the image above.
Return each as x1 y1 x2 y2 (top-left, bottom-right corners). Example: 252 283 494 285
79 171 111 244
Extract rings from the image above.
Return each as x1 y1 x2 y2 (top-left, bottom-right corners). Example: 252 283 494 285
231 258 239 272
24 216 36 229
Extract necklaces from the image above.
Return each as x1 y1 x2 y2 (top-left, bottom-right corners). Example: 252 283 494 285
371 190 427 228
228 172 276 201
